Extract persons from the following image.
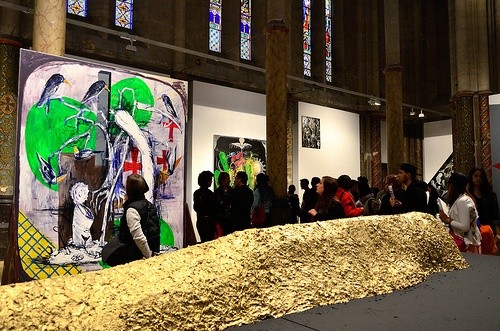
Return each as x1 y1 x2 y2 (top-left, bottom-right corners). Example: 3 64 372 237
467 168 500 243
283 184 301 224
439 173 482 255
213 172 232 236
229 172 254 232
194 171 218 242
299 168 452 224
118 174 161 263
389 163 428 214
252 173 273 228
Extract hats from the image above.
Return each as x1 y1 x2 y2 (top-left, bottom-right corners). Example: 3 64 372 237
401 163 417 178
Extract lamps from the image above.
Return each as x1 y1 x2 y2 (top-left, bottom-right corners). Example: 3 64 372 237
369 100 381 105
410 108 415 115
419 110 425 117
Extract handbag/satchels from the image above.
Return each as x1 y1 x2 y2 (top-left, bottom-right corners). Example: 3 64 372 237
102 237 129 267
214 223 223 239
450 232 466 254
252 203 266 227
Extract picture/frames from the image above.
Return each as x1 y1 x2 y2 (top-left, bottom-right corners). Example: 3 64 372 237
14 49 189 279
214 135 267 192
302 116 320 149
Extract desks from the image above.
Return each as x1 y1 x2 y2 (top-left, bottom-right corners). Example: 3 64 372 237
221 252 500 331
